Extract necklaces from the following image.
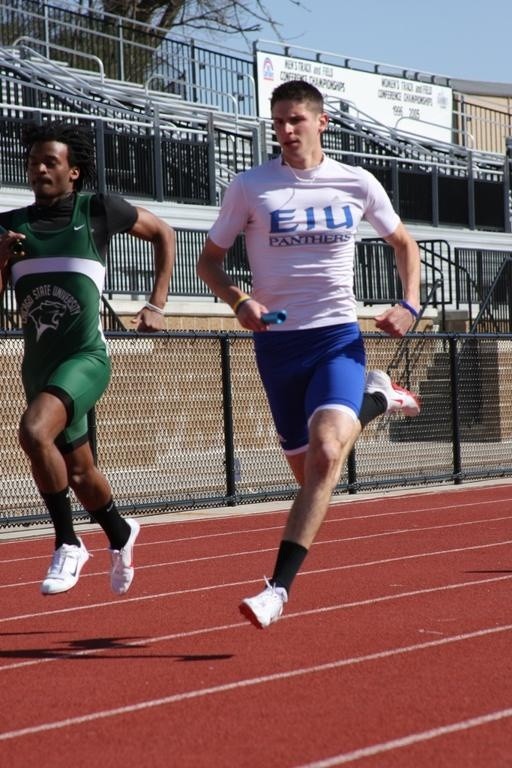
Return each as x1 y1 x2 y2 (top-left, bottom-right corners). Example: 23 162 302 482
283 158 323 184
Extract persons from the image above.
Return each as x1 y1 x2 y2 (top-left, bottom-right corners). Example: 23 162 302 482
0 122 182 600
192 76 422 633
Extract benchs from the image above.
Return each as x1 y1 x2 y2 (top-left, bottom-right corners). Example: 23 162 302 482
1 44 512 182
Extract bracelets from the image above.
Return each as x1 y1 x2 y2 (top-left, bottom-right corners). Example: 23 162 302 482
232 293 250 313
145 299 167 316
400 298 420 320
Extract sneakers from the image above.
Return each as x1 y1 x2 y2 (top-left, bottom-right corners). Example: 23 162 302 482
364 367 421 418
238 585 287 631
40 535 91 596
106 517 143 597
